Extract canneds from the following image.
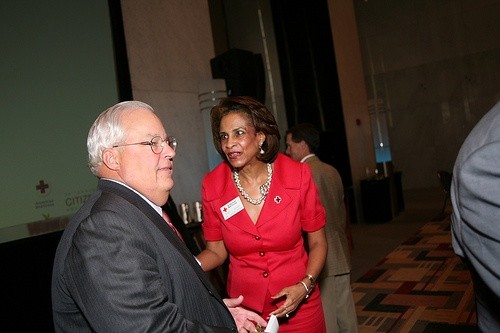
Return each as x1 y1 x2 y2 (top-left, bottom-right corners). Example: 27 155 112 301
193 201 203 222
180 202 192 224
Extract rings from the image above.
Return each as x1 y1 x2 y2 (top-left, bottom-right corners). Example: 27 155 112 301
286 314 290 318
254 325 263 333
285 308 289 312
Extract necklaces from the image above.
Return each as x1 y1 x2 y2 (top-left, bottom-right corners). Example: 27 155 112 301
233 162 273 204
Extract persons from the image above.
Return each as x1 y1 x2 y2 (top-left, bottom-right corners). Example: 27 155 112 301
52 101 280 333
284 123 359 333
192 96 328 333
449 99 500 333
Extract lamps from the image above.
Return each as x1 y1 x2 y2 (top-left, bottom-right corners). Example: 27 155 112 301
370 113 393 177
198 79 228 172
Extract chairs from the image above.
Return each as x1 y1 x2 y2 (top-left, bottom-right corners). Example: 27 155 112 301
438 171 452 212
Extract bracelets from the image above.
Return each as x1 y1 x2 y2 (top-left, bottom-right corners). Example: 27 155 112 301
304 274 315 293
298 280 310 299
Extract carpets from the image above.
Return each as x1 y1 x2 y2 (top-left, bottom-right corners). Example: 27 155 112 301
351 207 479 333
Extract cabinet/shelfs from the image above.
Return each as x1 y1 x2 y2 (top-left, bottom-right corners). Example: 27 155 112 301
360 172 405 224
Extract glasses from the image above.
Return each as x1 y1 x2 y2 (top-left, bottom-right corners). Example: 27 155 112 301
112 134 176 155
